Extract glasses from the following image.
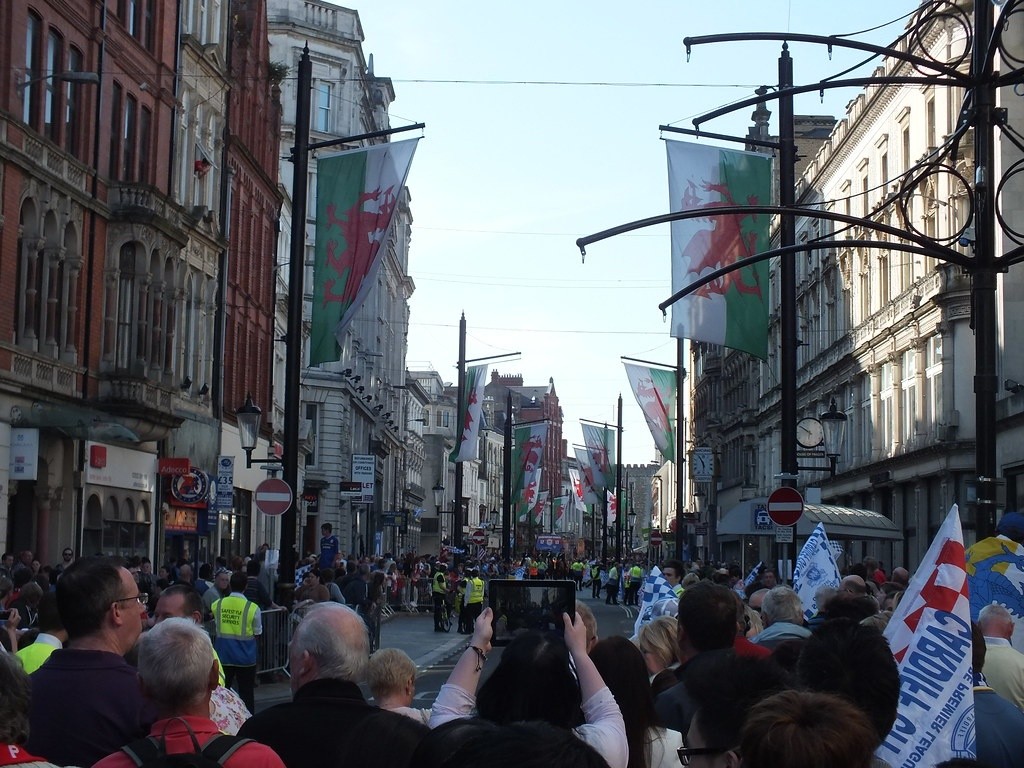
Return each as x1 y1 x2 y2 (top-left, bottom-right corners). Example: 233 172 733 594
116 592 148 604
678 746 721 766
662 572 673 577
64 554 72 557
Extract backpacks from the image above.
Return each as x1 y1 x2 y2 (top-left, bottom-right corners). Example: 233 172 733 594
122 717 256 768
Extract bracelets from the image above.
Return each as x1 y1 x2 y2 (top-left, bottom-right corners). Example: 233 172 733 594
465 643 488 671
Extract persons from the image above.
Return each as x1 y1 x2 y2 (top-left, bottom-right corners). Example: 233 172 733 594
935 512 1024 768
1 543 911 768
319 522 338 568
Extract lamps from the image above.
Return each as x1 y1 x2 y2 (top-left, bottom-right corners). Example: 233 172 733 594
374 405 383 411
341 368 352 377
390 385 407 389
1004 379 1020 392
387 420 394 426
382 413 390 419
356 386 364 394
351 352 384 357
410 418 426 422
184 376 209 395
272 260 315 272
350 375 361 383
391 426 399 432
362 395 372 402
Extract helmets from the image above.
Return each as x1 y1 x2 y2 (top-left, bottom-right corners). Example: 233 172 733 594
440 563 448 570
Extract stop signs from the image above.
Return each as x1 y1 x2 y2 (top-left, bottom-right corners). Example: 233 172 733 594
766 487 803 527
472 531 484 545
254 478 292 516
651 533 662 545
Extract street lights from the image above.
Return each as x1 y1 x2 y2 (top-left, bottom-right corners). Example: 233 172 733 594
615 508 637 563
432 480 463 566
774 397 847 588
490 506 510 564
676 490 706 561
234 392 300 667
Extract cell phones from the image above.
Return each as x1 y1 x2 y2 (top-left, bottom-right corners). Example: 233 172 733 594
0 610 11 621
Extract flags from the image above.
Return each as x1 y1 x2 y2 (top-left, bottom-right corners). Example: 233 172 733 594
295 563 312 589
510 423 616 527
664 139 774 362
871 502 978 768
732 560 762 598
308 139 419 368
793 523 843 622
628 564 678 636
515 566 526 580
623 364 677 466
449 364 486 463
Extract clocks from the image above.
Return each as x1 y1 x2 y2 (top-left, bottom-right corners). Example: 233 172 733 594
796 417 824 449
687 449 717 479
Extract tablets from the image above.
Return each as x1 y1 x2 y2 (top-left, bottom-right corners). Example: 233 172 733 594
488 579 576 647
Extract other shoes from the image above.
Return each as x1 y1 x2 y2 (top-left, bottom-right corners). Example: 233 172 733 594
439 627 448 633
457 628 463 634
592 595 596 599
464 629 472 634
606 601 613 605
596 594 601 598
614 602 620 605
434 627 440 632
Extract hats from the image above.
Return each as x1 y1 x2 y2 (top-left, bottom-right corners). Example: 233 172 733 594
14 567 34 587
214 567 228 579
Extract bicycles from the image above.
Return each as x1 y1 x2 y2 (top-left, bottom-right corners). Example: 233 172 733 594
439 590 455 633
